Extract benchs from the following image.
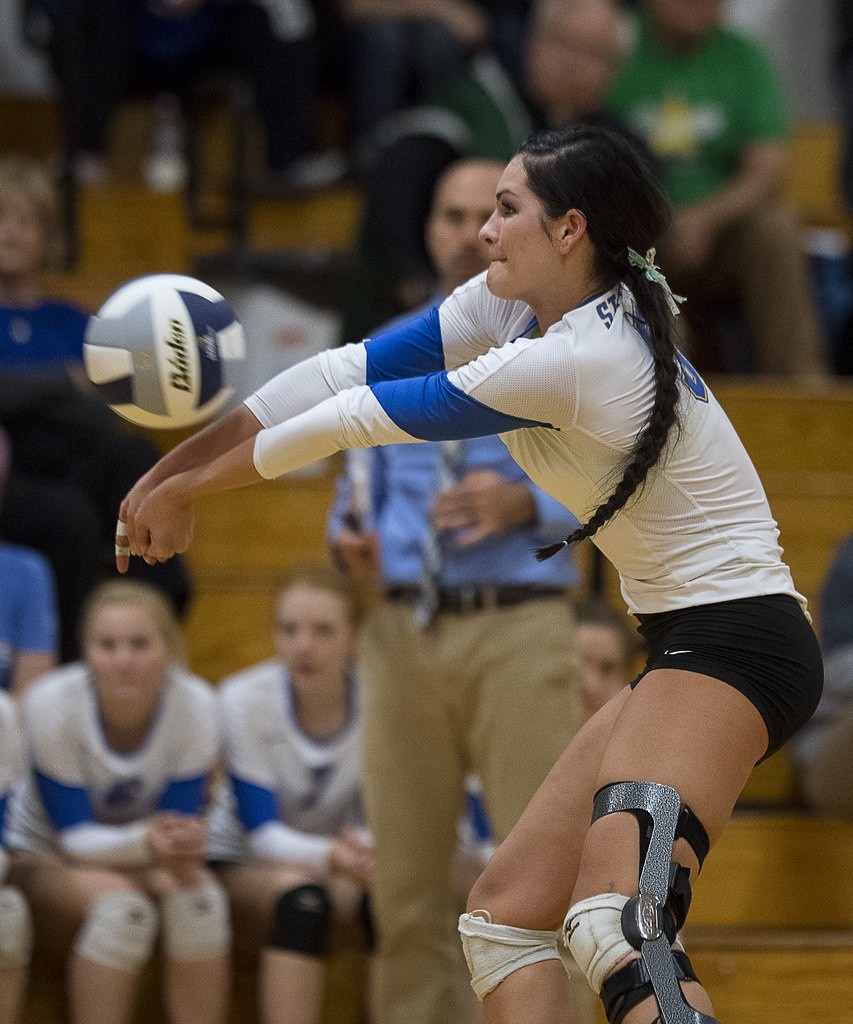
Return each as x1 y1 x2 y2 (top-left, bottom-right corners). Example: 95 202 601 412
35 109 853 1024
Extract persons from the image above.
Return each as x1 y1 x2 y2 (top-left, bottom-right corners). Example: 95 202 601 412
0 0 853 1023
116 120 824 1023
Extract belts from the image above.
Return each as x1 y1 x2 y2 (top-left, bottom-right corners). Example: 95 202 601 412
387 582 564 611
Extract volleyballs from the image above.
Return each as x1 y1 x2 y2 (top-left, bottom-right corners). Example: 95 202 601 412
81 273 247 431
